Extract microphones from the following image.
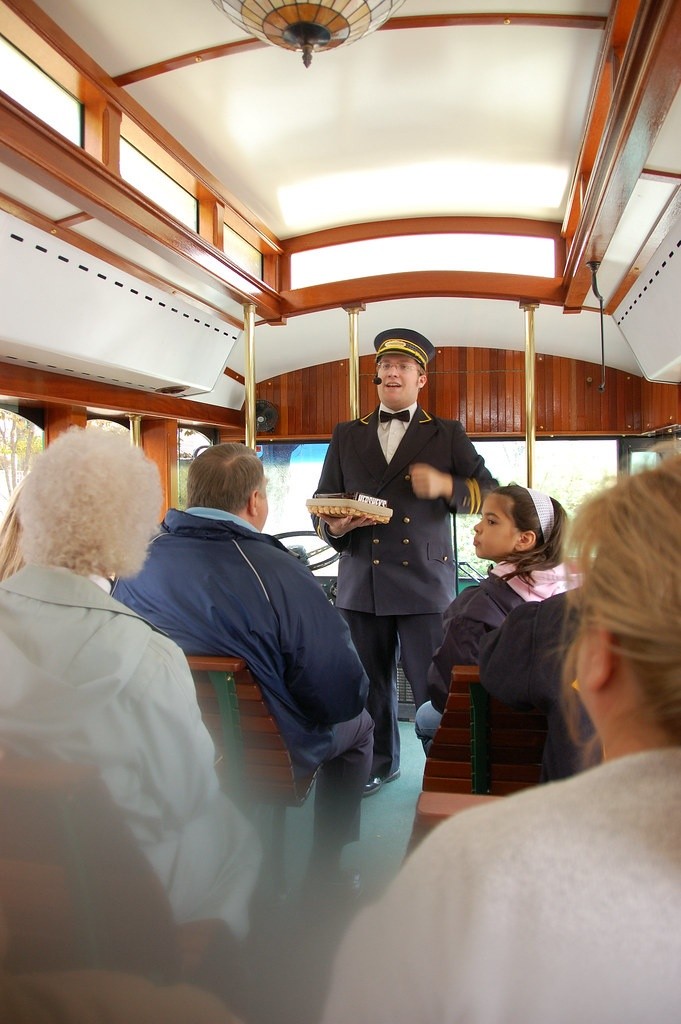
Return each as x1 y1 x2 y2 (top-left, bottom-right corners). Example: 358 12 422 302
372 374 382 384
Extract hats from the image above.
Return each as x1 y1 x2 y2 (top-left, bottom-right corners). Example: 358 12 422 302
374 328 435 374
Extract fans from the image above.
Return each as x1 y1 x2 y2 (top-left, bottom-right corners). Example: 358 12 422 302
253 399 278 436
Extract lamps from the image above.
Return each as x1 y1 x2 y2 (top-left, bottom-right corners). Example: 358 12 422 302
211 0 406 71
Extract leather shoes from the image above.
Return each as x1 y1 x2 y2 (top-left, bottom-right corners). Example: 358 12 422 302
363 762 401 796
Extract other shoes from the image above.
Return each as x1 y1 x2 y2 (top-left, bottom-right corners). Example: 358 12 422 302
315 872 363 904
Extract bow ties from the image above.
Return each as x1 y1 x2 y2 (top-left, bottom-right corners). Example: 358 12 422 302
380 409 410 423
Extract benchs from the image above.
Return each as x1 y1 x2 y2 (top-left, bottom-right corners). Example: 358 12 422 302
185 655 324 894
398 666 552 867
1 752 276 1024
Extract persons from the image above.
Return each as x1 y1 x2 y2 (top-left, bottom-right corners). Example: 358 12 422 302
324 454 681 1024
309 328 505 797
0 422 280 1023
109 440 375 923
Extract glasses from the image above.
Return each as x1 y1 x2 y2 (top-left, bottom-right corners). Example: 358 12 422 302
377 362 426 373
